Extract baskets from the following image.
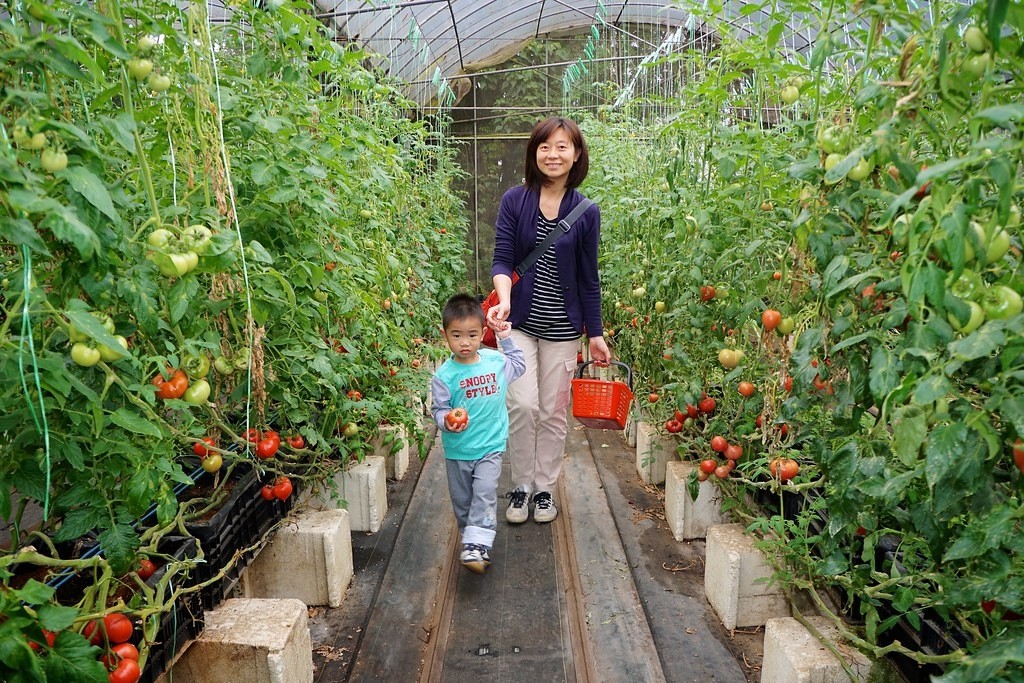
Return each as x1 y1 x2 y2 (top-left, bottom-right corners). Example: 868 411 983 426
570 360 633 431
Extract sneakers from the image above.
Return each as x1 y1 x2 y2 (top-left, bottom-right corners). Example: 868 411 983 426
530 492 558 522
505 490 531 524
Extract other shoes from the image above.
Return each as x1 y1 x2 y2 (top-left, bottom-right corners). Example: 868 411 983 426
458 544 486 575
481 549 491 568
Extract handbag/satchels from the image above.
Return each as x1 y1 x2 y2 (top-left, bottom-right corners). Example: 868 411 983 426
481 270 521 349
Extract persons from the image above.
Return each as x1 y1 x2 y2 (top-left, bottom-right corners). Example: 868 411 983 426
431 294 527 573
486 116 612 524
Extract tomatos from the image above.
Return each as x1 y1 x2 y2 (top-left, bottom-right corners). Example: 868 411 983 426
576 24 1024 627
7 33 470 683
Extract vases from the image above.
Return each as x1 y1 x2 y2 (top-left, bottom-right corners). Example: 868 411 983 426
737 448 1024 683
0 417 305 683
